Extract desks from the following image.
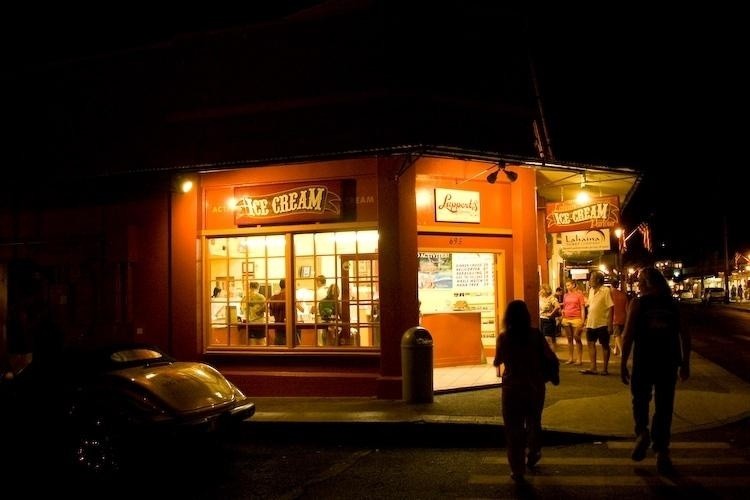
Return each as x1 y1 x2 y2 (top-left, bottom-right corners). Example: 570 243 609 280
237 321 333 349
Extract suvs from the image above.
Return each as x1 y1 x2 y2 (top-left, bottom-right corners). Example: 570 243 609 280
672 289 693 302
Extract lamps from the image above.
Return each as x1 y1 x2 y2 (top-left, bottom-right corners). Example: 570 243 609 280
485 158 519 185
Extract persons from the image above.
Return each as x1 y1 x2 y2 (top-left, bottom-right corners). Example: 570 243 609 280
310 275 329 346
268 280 304 345
211 286 223 298
538 269 630 377
620 266 693 468
492 300 561 480
318 283 341 345
239 282 268 347
730 283 737 298
737 283 743 299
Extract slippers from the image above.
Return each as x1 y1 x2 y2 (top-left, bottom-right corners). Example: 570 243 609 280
579 369 597 375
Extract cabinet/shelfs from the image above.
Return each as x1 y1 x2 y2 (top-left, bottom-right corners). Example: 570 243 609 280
471 301 498 357
367 307 484 371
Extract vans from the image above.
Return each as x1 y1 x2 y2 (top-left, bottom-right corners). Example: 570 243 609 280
701 288 718 303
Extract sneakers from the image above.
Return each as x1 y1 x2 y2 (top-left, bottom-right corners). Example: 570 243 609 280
631 433 651 461
527 452 541 469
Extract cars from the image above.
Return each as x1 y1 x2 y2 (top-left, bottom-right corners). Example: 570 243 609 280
707 289 725 302
2 336 258 479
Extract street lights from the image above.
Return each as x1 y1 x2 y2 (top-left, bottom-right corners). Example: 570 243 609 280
164 174 195 359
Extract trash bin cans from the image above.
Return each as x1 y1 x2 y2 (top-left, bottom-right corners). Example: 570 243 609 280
338 327 358 346
400 326 434 405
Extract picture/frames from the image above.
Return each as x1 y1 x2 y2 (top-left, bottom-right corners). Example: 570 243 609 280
299 266 312 279
242 274 256 289
241 261 254 273
